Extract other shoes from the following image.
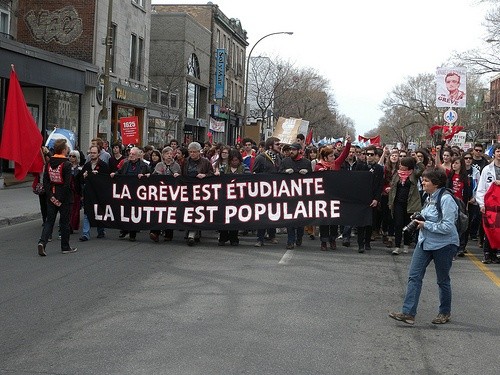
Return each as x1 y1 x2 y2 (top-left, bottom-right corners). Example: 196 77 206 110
188 238 200 246
38 242 47 256
482 252 499 265
343 242 351 247
97 232 105 237
383 236 392 247
330 240 337 251
218 239 239 245
321 241 327 251
79 237 89 240
285 239 302 250
63 248 78 253
150 234 160 242
119 232 126 238
254 239 263 246
131 238 135 242
456 247 468 257
358 243 371 253
393 244 409 253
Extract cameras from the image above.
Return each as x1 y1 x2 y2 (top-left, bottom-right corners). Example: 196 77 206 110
402 212 425 239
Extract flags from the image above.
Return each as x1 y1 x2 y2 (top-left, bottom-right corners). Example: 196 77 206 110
0 71 45 180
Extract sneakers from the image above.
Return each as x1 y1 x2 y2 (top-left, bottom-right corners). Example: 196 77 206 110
389 312 415 325
431 312 451 326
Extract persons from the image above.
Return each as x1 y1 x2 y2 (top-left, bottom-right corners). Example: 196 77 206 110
315 135 352 252
252 134 415 242
474 144 500 264
32 147 83 242
251 137 284 245
79 145 110 241
388 153 425 255
174 141 214 245
109 147 152 240
407 142 495 253
276 142 313 249
86 138 257 239
354 146 384 253
387 166 459 324
145 147 182 241
435 154 473 261
38 139 78 256
217 151 250 245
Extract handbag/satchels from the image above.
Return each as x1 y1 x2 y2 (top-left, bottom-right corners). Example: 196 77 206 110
32 183 45 195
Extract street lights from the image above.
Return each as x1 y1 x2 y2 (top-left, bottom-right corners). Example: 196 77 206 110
390 102 437 155
242 31 294 138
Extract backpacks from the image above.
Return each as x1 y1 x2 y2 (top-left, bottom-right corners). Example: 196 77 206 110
422 189 469 251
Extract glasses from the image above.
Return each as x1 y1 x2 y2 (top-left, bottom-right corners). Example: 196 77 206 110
475 148 482 153
275 143 280 146
69 154 76 158
463 157 473 159
366 153 374 157
360 152 365 156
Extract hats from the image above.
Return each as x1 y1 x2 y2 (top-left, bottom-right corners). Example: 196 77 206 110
289 143 301 150
